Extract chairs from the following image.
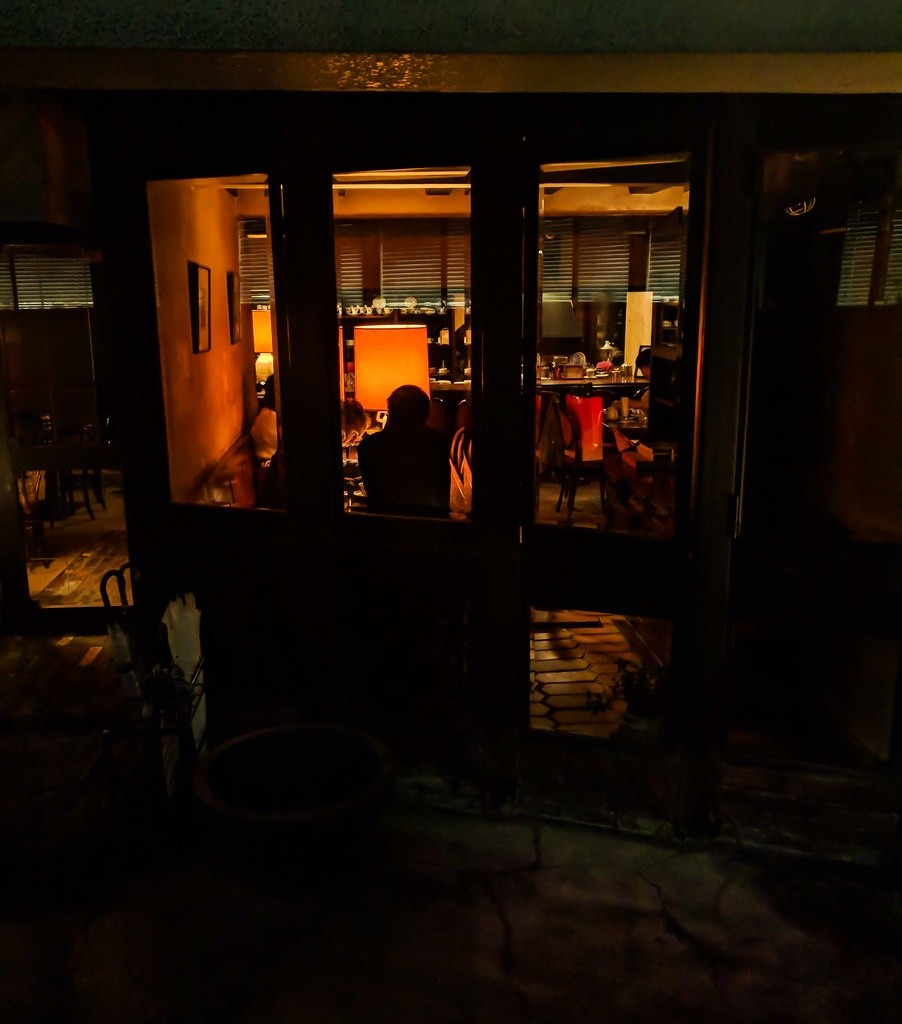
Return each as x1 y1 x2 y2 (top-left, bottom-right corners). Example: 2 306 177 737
540 387 606 526
69 416 111 521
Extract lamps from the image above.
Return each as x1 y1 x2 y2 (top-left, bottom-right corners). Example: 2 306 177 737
251 309 274 384
623 291 654 377
353 323 431 431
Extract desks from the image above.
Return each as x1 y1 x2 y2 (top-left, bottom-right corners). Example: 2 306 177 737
8 421 71 520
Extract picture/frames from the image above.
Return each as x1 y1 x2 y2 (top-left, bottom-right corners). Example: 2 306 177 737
192 263 212 354
228 271 241 344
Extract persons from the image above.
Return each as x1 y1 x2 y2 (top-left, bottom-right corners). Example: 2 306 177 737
611 349 650 410
253 375 381 501
358 385 477 513
